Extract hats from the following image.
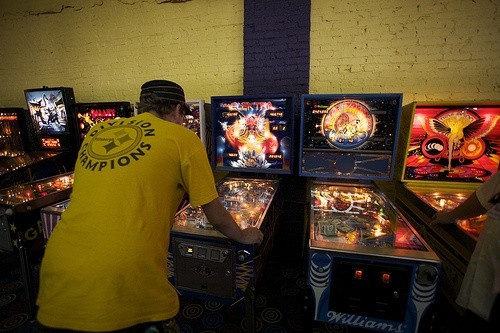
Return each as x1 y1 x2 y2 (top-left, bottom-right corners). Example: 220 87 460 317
140 80 192 115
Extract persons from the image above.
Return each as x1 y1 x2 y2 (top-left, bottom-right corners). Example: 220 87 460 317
429 162 500 333
35 78 265 333
